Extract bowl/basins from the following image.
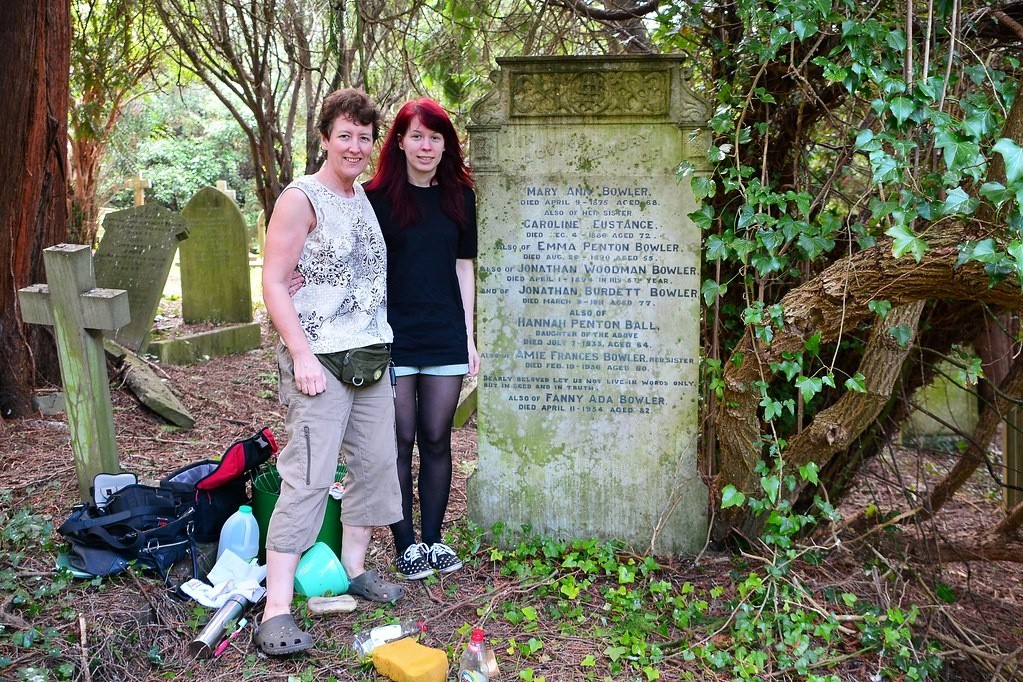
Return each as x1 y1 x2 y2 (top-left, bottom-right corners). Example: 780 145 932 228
292 543 348 601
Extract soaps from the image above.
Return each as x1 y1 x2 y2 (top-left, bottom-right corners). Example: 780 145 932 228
308 594 357 614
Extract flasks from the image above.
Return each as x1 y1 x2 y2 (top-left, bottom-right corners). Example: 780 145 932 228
190 595 248 660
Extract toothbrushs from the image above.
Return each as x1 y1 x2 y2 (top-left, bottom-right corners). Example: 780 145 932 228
214 617 248 657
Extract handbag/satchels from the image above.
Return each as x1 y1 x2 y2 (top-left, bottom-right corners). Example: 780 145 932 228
92 471 199 601
315 342 397 387
159 426 279 543
56 502 145 577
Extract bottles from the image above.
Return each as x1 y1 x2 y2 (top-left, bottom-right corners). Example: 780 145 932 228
457 629 490 682
354 621 426 659
216 505 258 565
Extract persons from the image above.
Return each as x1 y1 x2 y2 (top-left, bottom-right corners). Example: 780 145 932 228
287 99 480 582
253 89 405 655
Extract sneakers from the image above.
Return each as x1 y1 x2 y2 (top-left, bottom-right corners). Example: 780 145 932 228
395 543 434 580
424 542 463 574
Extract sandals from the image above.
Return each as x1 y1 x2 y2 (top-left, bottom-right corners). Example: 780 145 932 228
253 611 314 655
345 569 404 602
